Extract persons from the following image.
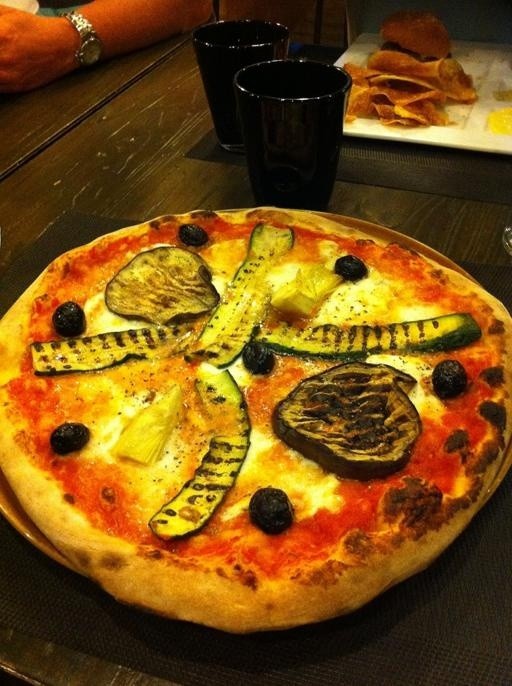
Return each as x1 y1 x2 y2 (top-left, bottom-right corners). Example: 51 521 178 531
0 0 214 93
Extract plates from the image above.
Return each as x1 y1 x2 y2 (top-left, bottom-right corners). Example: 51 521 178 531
331 31 512 157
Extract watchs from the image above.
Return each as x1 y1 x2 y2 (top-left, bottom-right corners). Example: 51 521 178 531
57 8 102 68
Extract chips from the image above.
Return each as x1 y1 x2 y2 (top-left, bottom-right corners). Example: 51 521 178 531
342 10 478 127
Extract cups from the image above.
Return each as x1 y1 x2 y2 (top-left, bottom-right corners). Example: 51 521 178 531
233 58 354 211
190 18 291 154
